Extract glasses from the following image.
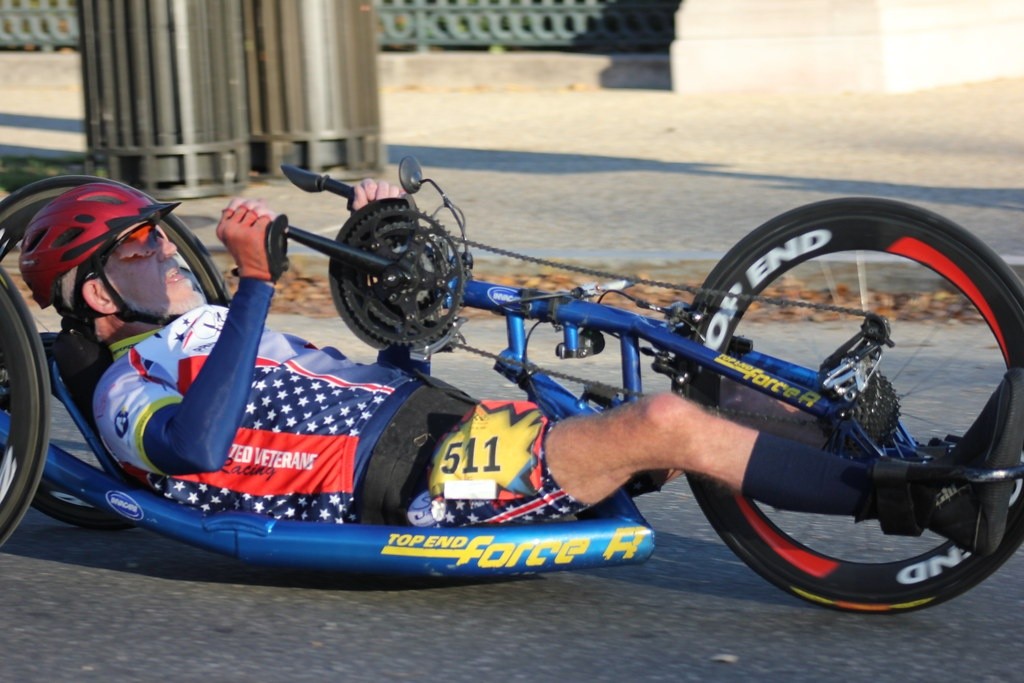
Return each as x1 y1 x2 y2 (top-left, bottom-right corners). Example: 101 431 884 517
91 224 157 280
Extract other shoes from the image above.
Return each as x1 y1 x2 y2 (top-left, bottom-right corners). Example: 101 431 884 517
853 363 1024 555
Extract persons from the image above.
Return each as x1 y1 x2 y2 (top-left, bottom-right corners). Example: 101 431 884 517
18 177 1024 558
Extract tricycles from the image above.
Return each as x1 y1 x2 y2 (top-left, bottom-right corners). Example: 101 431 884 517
0 156 1024 614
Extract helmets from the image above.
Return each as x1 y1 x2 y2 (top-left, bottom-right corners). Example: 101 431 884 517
19 183 182 309
